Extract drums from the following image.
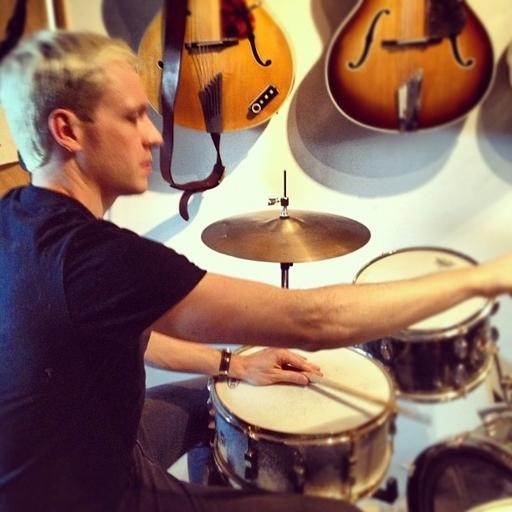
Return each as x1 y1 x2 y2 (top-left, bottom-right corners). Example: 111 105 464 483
208 345 397 502
408 417 512 512
352 246 498 402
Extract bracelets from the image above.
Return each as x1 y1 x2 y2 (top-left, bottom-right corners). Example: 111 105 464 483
217 348 234 376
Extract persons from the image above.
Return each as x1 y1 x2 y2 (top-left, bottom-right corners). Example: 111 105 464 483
0 27 512 512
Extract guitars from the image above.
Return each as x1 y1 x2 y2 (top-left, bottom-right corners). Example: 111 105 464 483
137 1 295 133
325 0 495 134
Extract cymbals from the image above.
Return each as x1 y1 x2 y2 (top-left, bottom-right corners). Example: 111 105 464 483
201 209 370 262
